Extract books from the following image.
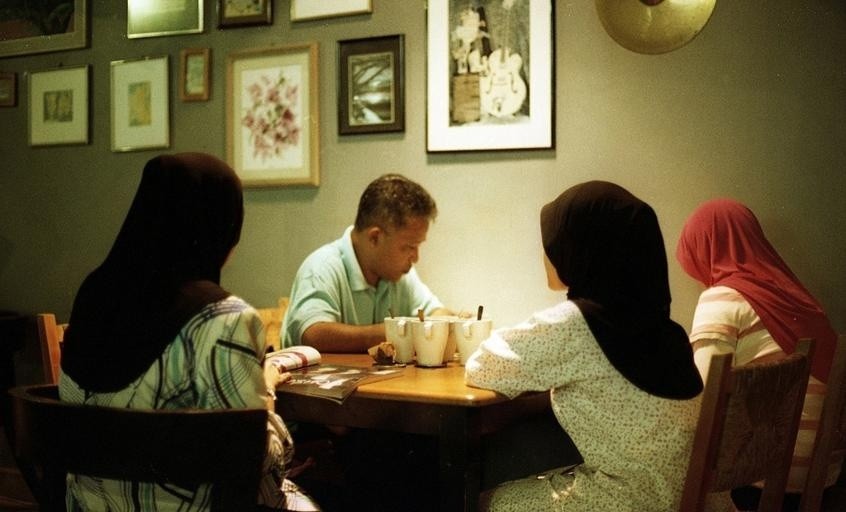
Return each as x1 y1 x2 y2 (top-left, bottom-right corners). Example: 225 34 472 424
264 345 403 405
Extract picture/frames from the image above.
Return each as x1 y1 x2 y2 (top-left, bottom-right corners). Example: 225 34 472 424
109 53 170 153
215 0 274 28
424 0 556 155
0 71 17 108
27 64 92 147
288 0 375 23
179 47 211 102
0 0 92 59
125 1 205 39
223 42 320 188
337 33 404 134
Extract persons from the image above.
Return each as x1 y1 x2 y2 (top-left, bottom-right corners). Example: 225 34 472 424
58 151 318 512
279 173 473 351
675 198 843 512
465 181 738 512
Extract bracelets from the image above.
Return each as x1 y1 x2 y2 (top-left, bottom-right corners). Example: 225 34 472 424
267 389 277 400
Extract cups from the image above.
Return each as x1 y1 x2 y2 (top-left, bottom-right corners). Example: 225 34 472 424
383 315 493 367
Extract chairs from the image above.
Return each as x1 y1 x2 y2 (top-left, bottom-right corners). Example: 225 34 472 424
11 384 268 512
801 334 846 512
37 312 71 387
679 338 814 510
251 296 288 350
